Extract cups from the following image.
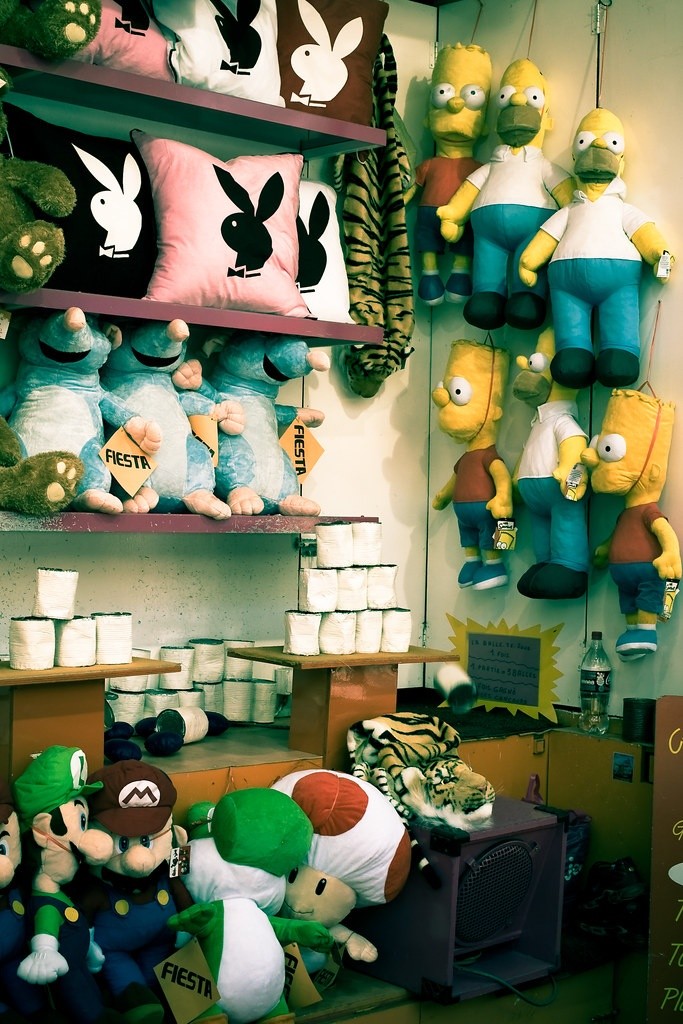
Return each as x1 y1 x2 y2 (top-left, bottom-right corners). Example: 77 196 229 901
622 696 657 743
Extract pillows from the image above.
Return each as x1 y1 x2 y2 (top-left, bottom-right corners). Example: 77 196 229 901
75 0 389 128
0 92 359 325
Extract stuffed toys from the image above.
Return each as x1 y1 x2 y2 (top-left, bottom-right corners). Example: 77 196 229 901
332 31 683 663
0 307 330 521
0 66 77 296
0 712 496 1024
0 0 102 67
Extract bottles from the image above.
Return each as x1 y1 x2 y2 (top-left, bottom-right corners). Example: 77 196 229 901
579 630 613 736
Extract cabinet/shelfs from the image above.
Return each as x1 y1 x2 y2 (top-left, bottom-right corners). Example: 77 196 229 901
0 41 387 534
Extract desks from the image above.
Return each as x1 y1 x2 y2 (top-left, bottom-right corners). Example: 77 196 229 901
1 657 183 787
225 644 461 756
